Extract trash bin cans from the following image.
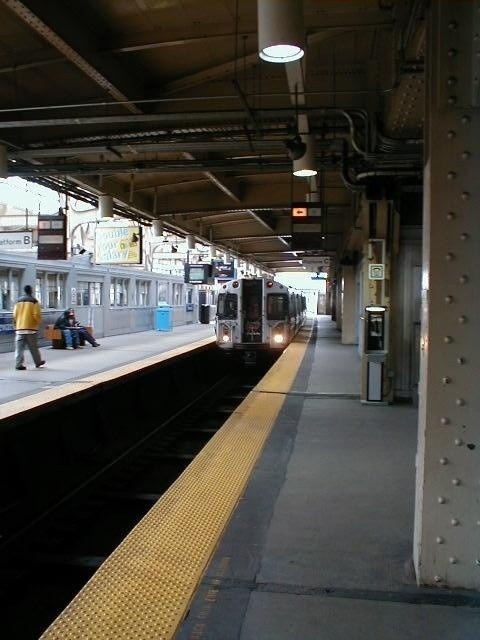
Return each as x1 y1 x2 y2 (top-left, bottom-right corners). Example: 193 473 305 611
154 305 173 332
199 303 210 324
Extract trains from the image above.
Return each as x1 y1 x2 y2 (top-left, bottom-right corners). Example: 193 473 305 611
214 274 310 367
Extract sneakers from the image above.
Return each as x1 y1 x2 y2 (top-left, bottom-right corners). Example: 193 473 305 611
66 345 75 350
35 360 46 368
93 342 101 347
76 344 84 349
16 365 28 371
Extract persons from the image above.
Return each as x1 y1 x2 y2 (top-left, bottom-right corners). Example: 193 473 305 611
67 308 100 347
12 285 46 370
53 310 83 350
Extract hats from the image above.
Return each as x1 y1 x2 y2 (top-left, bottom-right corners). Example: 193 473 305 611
64 308 75 317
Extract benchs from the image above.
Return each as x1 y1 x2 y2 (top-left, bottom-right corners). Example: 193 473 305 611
43 322 93 349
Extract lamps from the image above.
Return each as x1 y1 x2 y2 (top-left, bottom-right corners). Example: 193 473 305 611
255 0 309 66
292 143 318 178
185 233 264 278
152 218 164 236
97 193 114 219
132 233 140 243
170 239 177 253
